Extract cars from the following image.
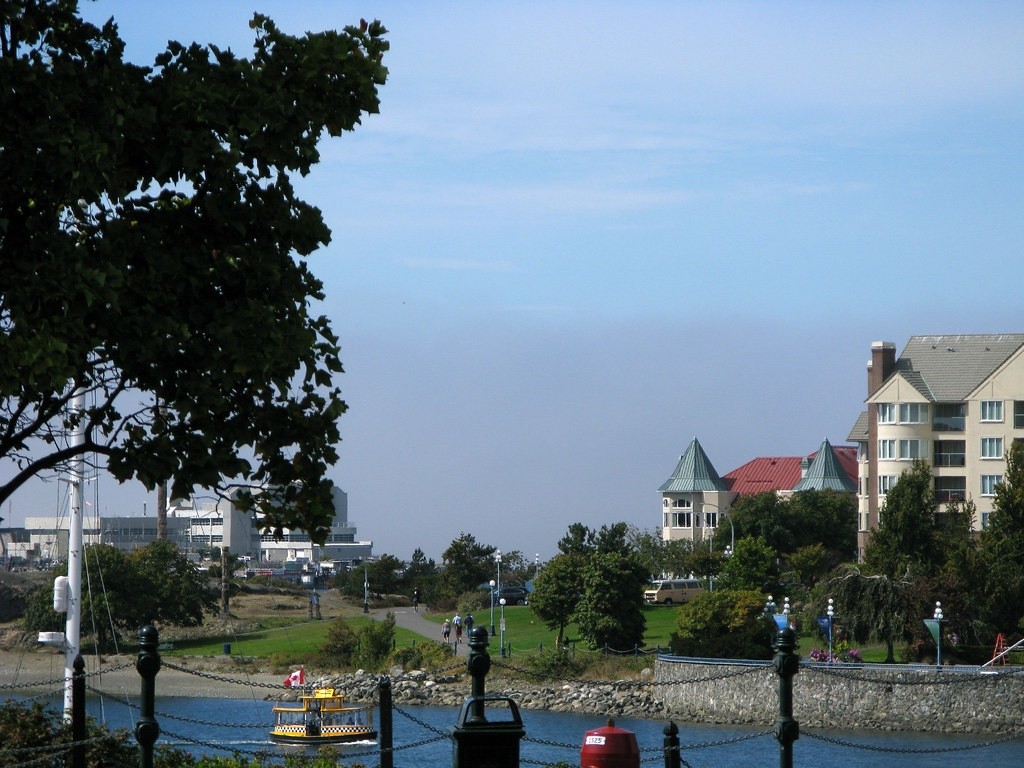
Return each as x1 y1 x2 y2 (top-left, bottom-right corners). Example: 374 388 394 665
487 587 529 607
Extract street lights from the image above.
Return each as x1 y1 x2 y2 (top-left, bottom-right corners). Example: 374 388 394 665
498 598 507 654
490 580 497 636
934 601 944 666
535 552 541 576
494 549 505 597
684 510 712 596
700 502 734 556
827 598 834 663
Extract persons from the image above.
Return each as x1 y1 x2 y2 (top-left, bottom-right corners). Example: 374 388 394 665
441 612 476 644
414 597 419 613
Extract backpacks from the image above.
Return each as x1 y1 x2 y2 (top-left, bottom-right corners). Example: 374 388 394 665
456 627 462 635
466 617 473 626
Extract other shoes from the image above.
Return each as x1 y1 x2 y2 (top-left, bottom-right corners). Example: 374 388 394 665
460 638 462 644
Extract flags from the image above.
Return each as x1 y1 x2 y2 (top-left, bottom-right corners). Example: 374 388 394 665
284 668 305 687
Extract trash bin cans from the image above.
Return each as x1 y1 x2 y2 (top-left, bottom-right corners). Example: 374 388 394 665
445 696 528 768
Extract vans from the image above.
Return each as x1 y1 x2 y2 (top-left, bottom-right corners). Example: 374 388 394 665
643 579 706 606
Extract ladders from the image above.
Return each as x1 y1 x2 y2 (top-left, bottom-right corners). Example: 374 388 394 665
990 633 1011 671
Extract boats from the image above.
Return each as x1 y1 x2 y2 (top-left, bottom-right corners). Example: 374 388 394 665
268 687 379 743
